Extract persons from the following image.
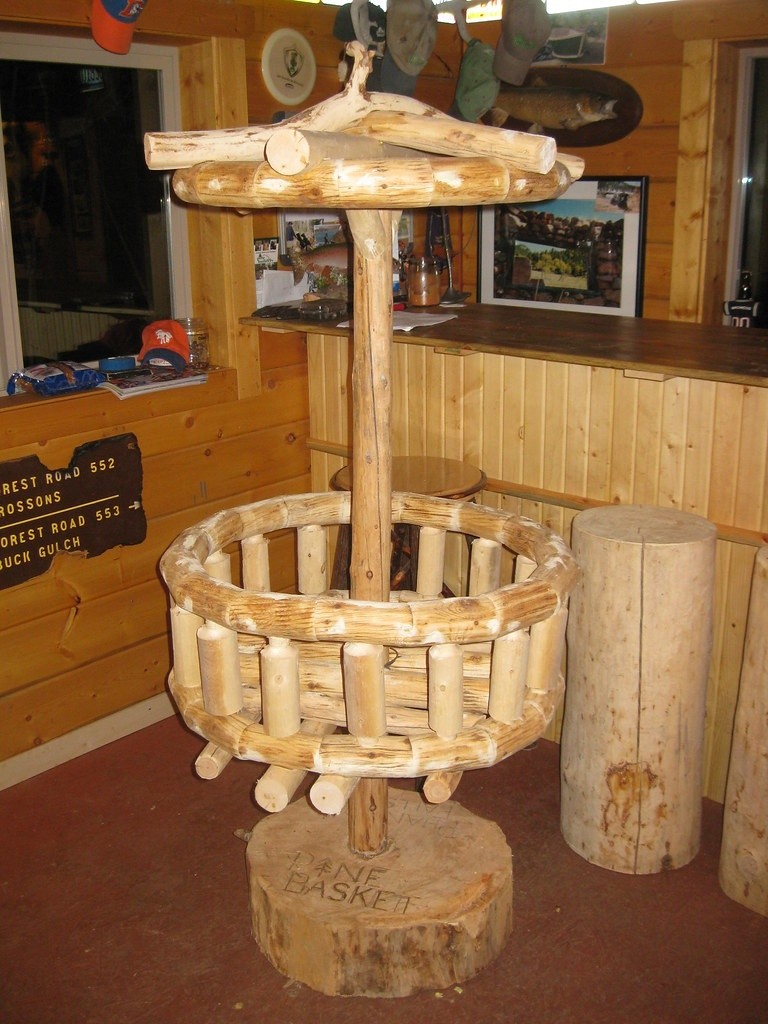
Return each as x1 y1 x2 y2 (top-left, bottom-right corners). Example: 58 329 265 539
324 234 329 244
254 238 277 252
617 188 632 210
610 194 618 206
286 222 298 241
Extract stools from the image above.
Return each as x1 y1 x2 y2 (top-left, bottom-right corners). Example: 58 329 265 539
327 458 493 600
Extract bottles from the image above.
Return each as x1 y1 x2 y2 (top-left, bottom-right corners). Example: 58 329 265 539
176 315 211 371
406 257 441 308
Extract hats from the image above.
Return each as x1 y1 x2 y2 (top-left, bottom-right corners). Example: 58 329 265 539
448 38 501 122
138 319 191 373
90 0 147 53
381 0 439 94
492 0 552 85
333 1 387 49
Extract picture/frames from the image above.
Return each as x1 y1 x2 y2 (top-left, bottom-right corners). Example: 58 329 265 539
472 174 648 317
